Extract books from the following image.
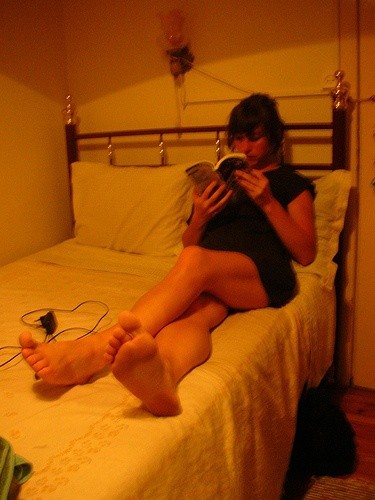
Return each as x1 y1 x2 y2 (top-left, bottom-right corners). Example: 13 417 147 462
184 152 248 206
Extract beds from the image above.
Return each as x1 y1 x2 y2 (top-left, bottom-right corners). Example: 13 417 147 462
0 69 352 500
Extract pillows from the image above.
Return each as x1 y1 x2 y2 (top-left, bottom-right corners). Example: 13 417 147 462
291 168 353 291
70 160 215 256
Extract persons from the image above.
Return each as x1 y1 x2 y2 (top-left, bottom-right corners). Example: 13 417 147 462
18 93 317 417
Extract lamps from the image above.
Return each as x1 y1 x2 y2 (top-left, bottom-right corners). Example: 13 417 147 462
159 9 196 88
